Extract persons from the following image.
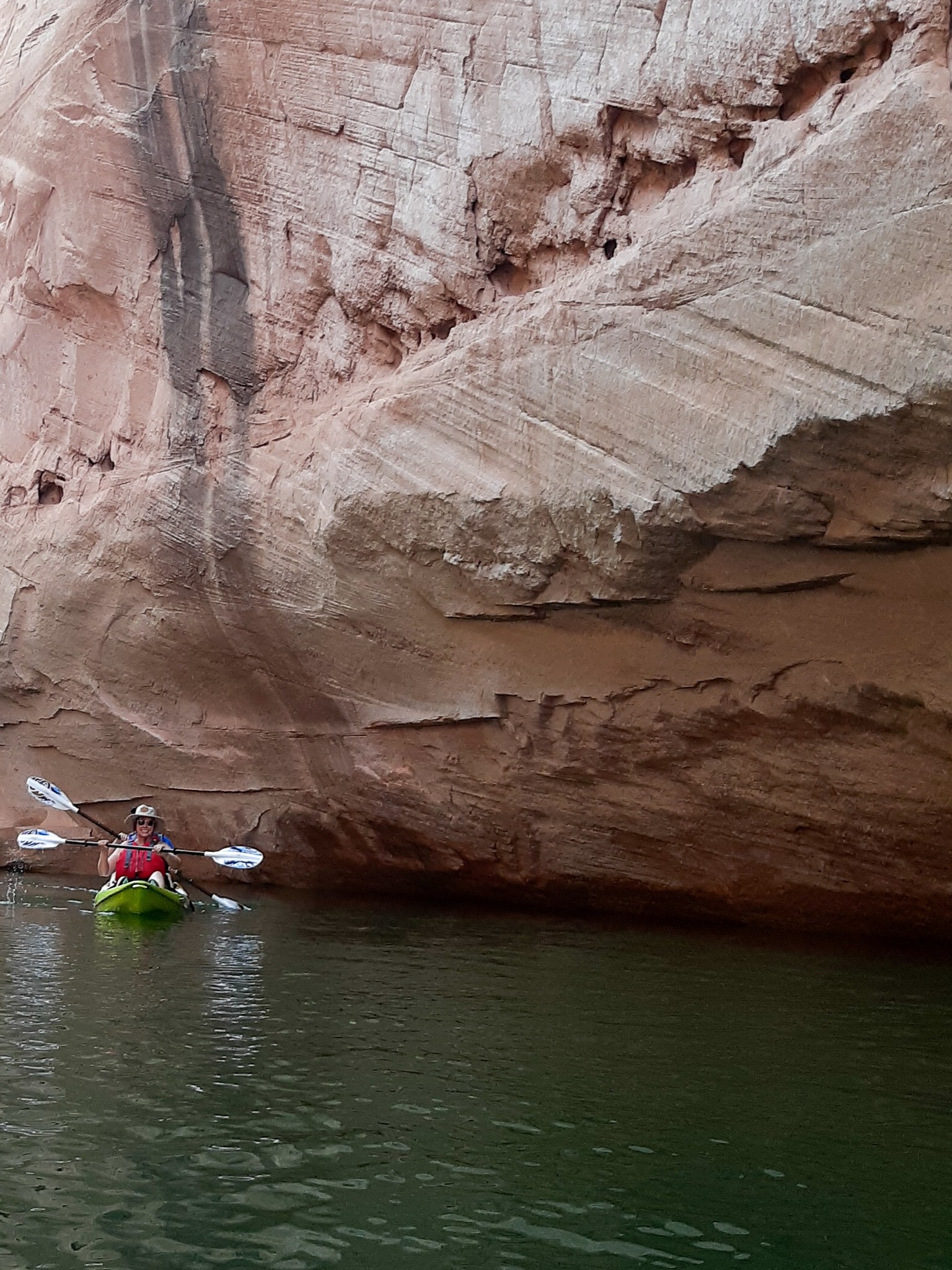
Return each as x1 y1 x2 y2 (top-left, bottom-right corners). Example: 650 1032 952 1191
97 803 180 891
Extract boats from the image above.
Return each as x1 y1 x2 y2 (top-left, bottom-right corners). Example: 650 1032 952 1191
92 879 192 920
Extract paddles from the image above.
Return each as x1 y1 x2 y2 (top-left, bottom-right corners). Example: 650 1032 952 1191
26 775 252 911
16 828 265 870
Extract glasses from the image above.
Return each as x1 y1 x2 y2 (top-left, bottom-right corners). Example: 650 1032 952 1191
136 819 155 826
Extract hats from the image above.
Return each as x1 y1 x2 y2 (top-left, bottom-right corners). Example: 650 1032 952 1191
124 804 165 830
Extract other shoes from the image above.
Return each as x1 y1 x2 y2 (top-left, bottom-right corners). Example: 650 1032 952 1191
173 882 180 889
148 879 159 886
116 876 129 887
